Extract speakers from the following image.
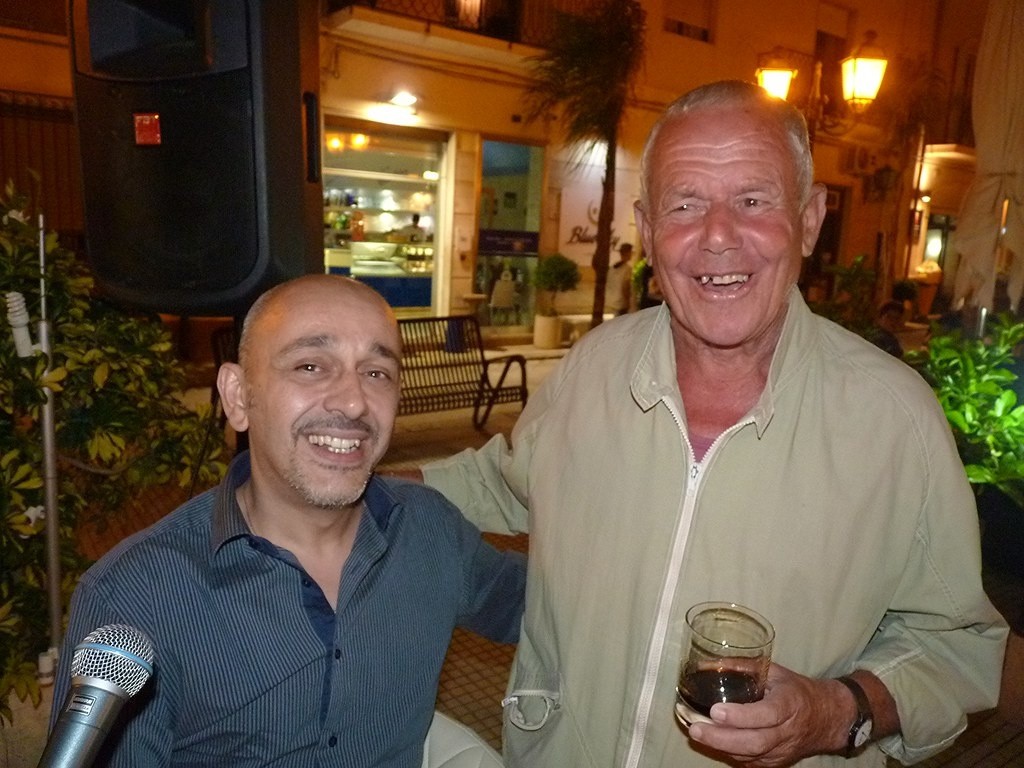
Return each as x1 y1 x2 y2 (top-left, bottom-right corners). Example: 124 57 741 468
66 0 325 319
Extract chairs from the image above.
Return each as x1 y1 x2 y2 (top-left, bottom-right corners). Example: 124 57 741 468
487 280 516 325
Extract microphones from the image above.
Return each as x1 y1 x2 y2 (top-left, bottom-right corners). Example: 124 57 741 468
35 624 154 768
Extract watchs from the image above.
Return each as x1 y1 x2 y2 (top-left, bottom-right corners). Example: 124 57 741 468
833 676 874 758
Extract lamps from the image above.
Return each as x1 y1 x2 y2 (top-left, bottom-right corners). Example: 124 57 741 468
867 160 900 206
388 82 421 114
917 189 934 206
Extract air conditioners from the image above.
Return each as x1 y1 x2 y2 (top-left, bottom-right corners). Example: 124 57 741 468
847 144 878 175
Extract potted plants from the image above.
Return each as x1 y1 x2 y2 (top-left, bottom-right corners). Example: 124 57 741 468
531 248 583 349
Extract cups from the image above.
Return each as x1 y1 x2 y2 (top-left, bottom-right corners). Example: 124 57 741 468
673 601 776 728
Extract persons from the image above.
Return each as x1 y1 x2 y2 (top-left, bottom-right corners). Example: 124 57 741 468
404 215 427 242
378 80 1010 768
48 275 527 768
614 243 633 269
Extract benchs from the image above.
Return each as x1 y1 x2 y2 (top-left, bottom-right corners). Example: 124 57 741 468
393 309 530 442
